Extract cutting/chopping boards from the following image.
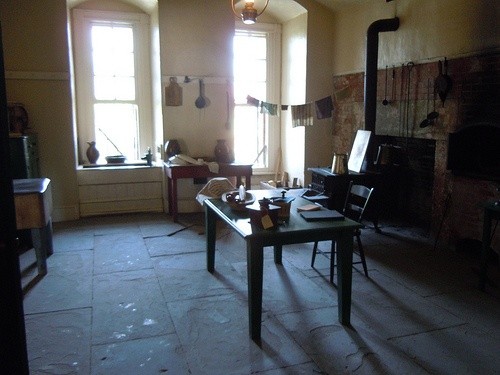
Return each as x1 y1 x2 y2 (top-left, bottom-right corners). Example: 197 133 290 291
164 76 183 106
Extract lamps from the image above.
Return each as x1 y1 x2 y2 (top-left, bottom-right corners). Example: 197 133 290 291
231 0 270 24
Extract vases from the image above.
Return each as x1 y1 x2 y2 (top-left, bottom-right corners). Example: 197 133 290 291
214 139 229 162
86 140 99 163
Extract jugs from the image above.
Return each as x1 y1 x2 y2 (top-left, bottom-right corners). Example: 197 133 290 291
331 152 348 175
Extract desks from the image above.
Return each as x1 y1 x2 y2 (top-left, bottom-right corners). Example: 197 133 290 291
202 189 366 341
13 177 54 275
478 202 500 292
259 180 302 190
163 161 253 222
307 165 380 232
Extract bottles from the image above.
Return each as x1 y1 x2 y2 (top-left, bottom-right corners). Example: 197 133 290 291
87 141 100 163
214 139 228 163
165 139 180 157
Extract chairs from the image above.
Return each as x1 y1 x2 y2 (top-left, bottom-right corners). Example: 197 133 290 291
310 180 375 284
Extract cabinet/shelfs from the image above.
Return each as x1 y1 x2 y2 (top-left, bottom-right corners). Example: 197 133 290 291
10 131 41 247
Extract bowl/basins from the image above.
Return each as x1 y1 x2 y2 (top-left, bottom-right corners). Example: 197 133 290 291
222 191 255 210
105 156 126 163
